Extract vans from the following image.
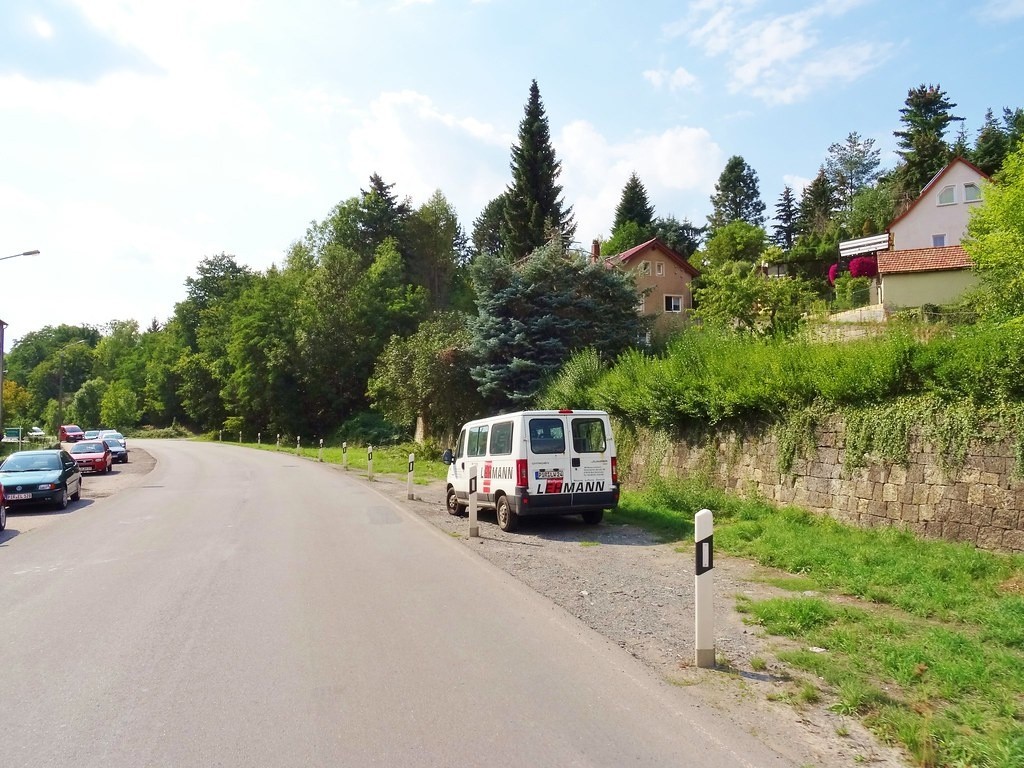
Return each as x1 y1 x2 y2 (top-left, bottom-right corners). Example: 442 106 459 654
443 409 621 533
59 424 85 443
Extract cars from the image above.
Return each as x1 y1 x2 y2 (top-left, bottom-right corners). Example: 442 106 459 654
0 483 10 532
97 429 118 441
69 440 113 475
100 439 129 463
27 427 46 439
0 449 83 511
82 430 100 441
1 430 20 442
102 433 126 448
